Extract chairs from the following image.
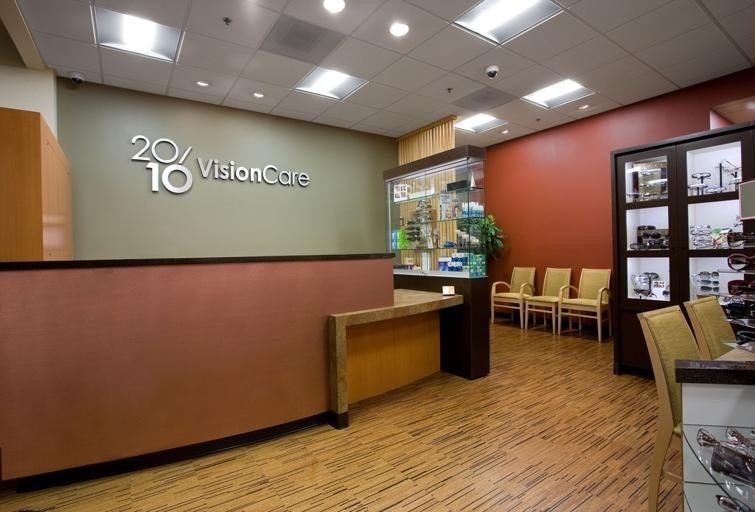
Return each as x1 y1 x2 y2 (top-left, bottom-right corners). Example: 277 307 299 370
636 286 738 512
490 262 615 341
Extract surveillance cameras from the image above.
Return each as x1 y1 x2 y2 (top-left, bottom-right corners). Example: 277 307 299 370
486 65 499 80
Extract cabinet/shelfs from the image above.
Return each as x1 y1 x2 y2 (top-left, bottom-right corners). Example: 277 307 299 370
610 123 750 375
385 143 486 276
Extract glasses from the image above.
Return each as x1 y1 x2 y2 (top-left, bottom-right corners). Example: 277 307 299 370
727 223 755 247
730 319 755 345
698 280 719 286
691 172 711 179
700 286 720 292
714 158 742 184
690 224 717 249
728 253 755 272
726 304 755 320
629 239 669 251
705 186 727 193
643 232 661 238
638 225 656 230
687 184 708 189
715 495 754 512
662 289 670 296
642 272 658 279
696 271 719 280
633 273 657 299
728 279 755 295
723 297 744 304
696 426 755 488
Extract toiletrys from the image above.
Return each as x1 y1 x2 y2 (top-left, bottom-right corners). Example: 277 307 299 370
451 197 485 217
455 230 479 249
400 215 403 225
434 233 438 248
